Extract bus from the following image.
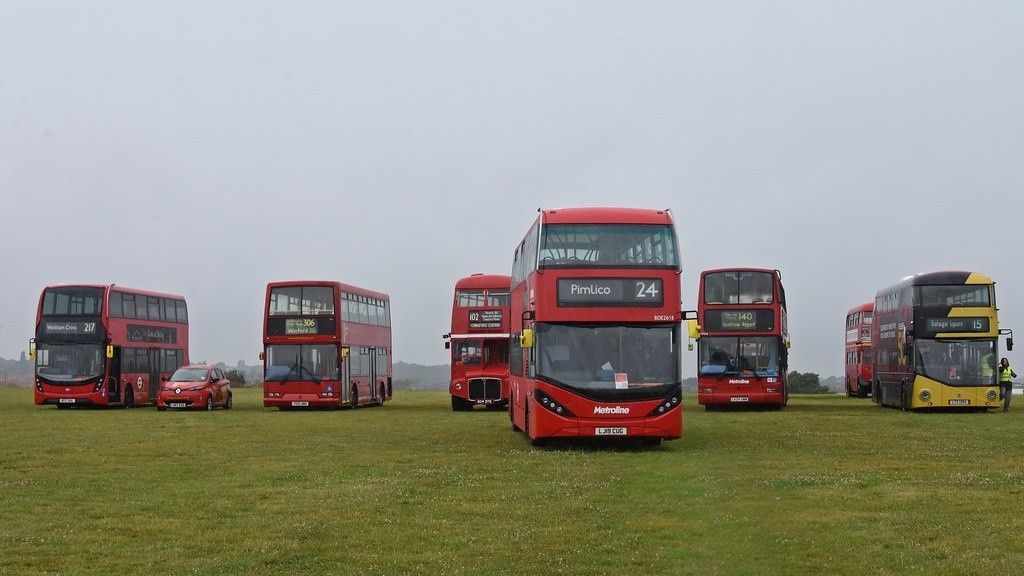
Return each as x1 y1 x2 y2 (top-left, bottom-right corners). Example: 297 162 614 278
688 265 791 411
259 280 393 409
870 269 1013 412
28 282 189 410
510 206 701 451
442 274 515 411
843 302 875 398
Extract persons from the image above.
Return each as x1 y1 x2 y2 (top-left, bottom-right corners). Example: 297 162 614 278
979 347 996 385
999 358 1017 413
951 345 964 365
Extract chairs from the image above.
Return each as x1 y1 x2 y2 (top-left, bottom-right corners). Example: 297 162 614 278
317 311 333 315
272 312 300 315
753 298 773 303
542 257 662 264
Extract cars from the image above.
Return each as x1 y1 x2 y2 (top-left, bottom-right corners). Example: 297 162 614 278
156 365 233 412
